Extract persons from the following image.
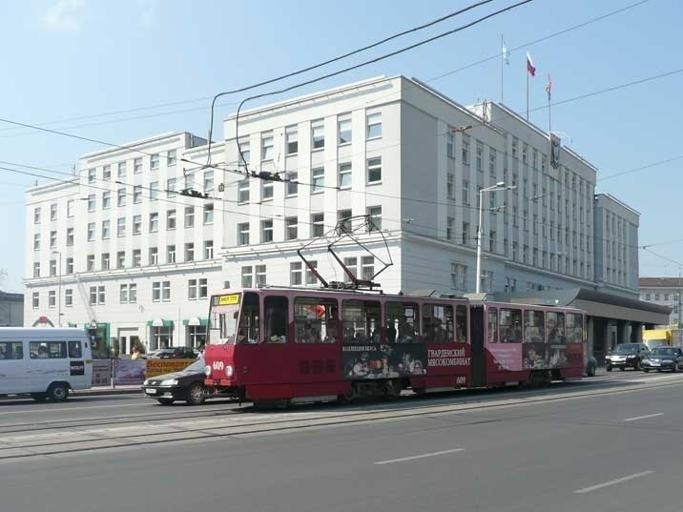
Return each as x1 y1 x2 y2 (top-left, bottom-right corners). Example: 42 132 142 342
294 318 466 343
0 345 80 359
129 346 141 360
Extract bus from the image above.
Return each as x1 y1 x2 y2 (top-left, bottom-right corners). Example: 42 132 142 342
204 287 585 409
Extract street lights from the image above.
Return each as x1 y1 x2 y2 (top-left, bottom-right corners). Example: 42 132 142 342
474 181 518 294
51 249 61 329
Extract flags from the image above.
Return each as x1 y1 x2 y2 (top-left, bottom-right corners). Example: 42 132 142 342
525 52 536 78
543 73 553 100
501 39 511 67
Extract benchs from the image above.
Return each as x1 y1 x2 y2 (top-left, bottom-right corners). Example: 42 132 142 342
111 378 144 389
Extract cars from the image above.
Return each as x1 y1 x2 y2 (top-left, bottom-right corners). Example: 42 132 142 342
156 346 198 359
140 357 246 405
586 343 682 378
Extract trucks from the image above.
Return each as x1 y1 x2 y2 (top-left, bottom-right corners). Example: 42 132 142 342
642 328 683 350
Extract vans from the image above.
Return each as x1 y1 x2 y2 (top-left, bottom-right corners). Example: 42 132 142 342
0 329 94 402
